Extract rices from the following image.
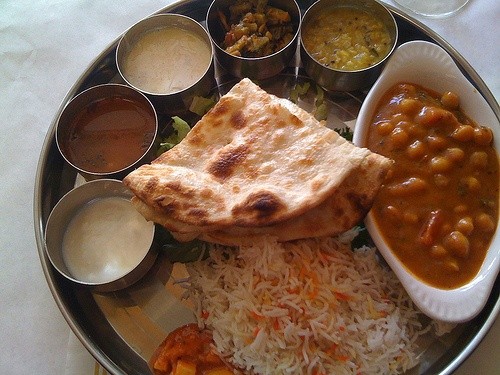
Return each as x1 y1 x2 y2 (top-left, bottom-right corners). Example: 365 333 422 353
166 225 458 375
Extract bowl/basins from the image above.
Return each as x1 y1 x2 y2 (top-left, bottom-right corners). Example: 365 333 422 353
43 2 402 292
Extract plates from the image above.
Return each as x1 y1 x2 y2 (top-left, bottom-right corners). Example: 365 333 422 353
353 37 495 368
33 1 499 375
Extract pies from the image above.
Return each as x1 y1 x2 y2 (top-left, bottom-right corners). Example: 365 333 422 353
124 78 394 243
